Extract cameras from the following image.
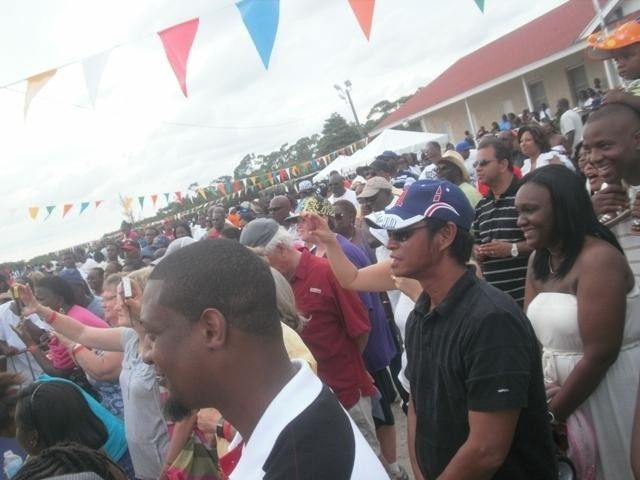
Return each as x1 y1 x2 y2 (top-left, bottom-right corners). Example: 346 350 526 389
120 277 132 300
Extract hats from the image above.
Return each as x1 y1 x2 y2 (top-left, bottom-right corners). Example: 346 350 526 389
357 142 473 230
587 18 640 60
239 217 279 248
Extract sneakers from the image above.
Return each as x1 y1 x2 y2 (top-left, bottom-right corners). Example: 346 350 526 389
388 463 408 480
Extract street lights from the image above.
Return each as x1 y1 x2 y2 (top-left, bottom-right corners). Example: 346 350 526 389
332 80 361 128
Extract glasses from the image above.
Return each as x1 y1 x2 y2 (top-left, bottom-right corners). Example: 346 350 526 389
196 182 349 233
60 221 187 282
386 225 443 242
474 160 506 167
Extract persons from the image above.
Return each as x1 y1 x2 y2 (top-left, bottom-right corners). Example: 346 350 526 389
1 19 639 480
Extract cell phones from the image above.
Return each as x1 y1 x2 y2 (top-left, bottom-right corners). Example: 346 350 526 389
11 285 23 312
